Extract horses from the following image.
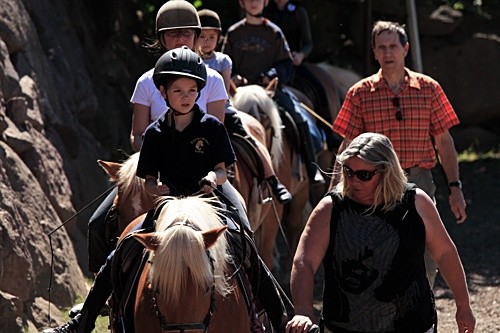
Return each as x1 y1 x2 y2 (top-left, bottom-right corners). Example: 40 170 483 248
228 85 330 274
303 61 362 127
97 109 284 271
109 195 251 333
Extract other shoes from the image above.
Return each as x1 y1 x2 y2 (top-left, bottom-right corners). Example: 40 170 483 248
326 132 344 152
68 303 84 318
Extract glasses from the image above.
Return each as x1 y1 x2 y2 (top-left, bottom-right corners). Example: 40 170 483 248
342 162 380 182
392 96 403 122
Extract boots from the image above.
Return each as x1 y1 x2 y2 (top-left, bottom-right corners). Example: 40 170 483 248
267 174 292 204
295 120 326 188
43 313 96 333
278 314 320 333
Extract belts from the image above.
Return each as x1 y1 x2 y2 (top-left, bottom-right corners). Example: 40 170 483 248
404 165 423 177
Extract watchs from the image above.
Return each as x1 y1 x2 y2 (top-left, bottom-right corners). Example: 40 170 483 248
449 180 461 188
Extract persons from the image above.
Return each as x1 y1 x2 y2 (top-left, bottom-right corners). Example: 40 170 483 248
69 0 254 320
285 132 476 333
263 0 341 151
195 9 293 205
220 0 326 187
328 21 466 289
50 43 321 333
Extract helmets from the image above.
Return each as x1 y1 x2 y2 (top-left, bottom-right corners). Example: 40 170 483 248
156 0 202 42
195 9 221 41
153 45 208 91
